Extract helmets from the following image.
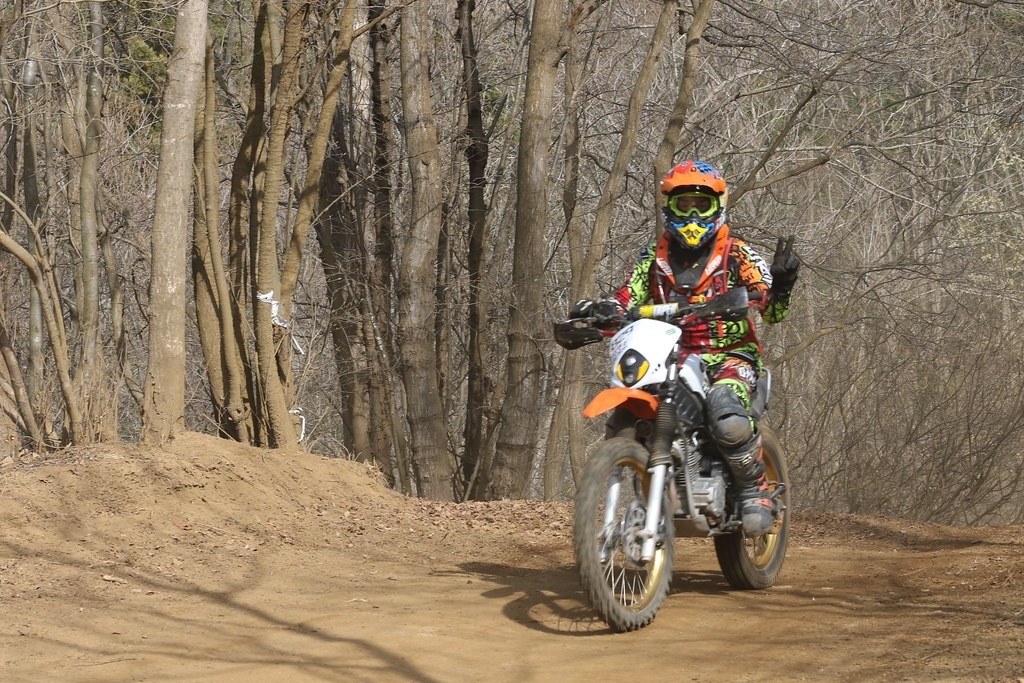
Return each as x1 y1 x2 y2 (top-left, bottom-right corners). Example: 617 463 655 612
659 160 729 249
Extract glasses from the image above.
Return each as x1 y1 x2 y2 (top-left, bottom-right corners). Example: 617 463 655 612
670 191 718 217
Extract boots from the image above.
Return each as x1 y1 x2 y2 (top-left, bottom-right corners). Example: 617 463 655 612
717 426 775 538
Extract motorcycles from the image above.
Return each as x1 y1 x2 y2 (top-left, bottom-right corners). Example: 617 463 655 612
554 287 790 633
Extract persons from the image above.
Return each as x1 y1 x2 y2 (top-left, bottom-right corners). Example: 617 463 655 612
567 160 799 538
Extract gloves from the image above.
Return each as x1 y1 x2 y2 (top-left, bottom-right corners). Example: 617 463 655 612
769 235 801 293
567 298 596 318
